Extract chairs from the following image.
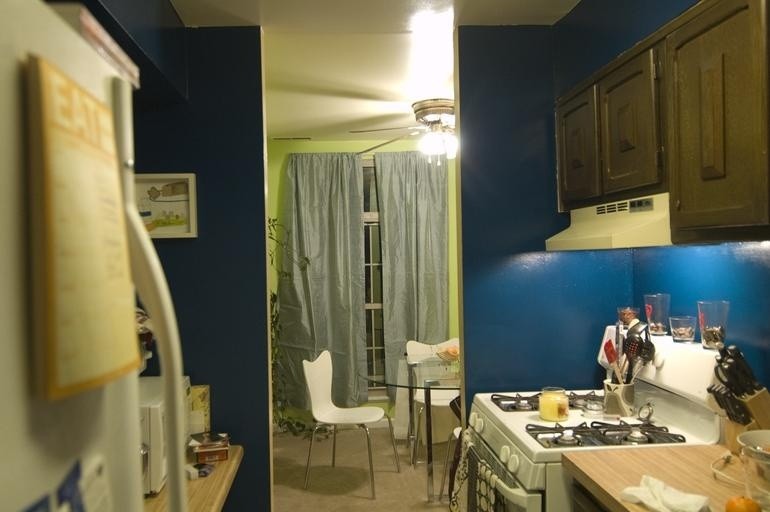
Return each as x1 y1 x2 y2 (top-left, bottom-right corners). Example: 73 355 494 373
302 349 400 500
440 427 462 502
407 338 459 468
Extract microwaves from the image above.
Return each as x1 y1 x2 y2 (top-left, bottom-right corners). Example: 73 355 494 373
139 376 191 497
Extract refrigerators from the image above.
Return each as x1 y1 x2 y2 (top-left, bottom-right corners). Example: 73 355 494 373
0 4 190 511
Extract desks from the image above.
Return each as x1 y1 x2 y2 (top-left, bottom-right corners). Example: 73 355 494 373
561 444 766 512
142 444 243 512
357 357 461 506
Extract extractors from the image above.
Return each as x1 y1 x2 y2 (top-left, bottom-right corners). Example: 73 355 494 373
544 193 671 250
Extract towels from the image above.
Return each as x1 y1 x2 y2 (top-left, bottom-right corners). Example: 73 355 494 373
451 430 504 512
621 474 710 512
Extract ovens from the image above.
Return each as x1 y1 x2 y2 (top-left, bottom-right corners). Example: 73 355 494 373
463 426 545 511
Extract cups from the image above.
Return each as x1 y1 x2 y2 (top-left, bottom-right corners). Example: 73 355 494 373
697 300 729 348
617 306 641 328
644 294 670 335
603 379 634 416
668 316 697 341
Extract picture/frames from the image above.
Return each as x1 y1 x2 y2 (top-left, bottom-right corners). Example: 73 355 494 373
133 173 197 240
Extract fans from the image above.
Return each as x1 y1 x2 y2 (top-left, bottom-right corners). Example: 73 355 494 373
348 97 459 168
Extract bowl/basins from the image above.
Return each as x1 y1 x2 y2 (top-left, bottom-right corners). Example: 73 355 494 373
737 429 770 512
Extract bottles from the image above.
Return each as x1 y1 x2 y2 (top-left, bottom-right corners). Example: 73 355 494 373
539 386 569 422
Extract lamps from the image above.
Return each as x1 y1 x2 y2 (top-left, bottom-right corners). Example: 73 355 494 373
419 121 458 165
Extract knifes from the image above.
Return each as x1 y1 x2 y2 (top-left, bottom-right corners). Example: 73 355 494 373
707 341 762 426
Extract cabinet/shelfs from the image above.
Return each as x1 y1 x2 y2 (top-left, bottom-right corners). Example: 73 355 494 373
552 25 668 213
661 0 770 244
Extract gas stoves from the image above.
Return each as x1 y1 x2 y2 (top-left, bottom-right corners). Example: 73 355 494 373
467 385 686 491
